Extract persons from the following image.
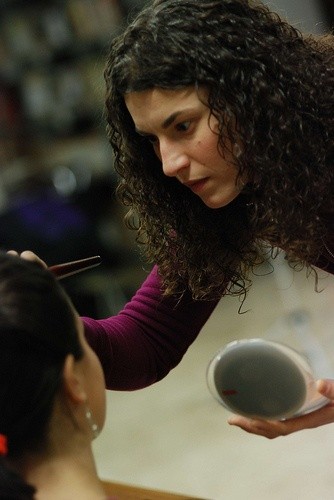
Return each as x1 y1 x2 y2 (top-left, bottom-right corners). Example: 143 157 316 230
1 248 202 500
7 1 334 439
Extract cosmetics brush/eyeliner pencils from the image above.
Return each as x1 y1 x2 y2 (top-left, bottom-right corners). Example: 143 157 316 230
47 255 102 281
